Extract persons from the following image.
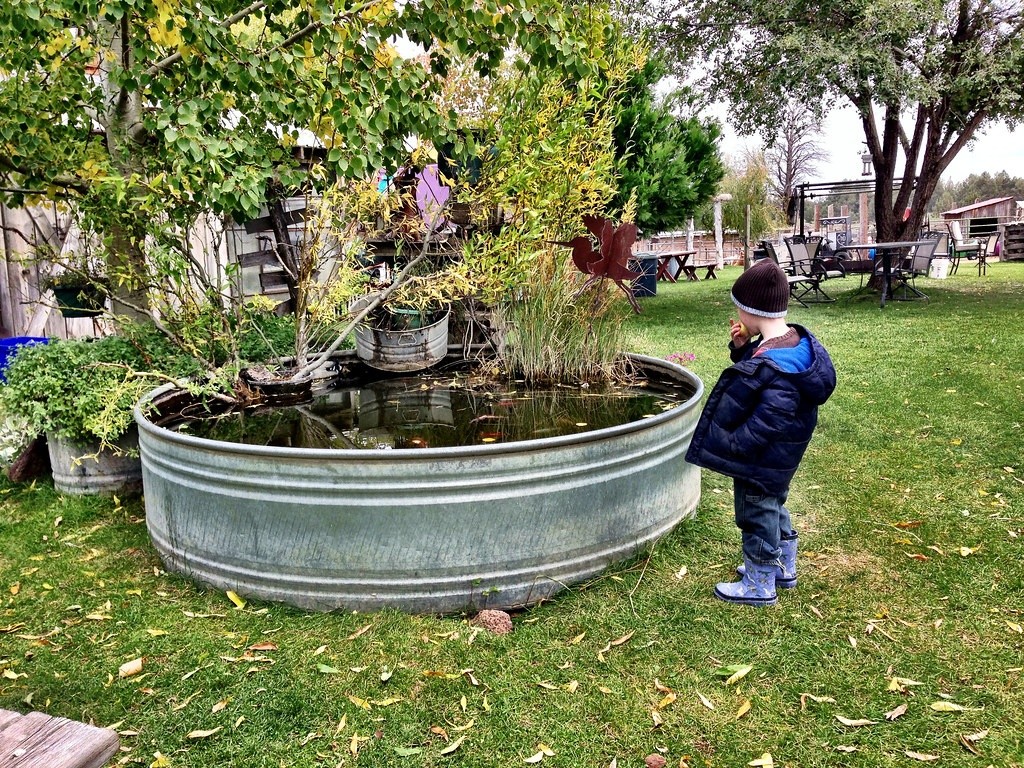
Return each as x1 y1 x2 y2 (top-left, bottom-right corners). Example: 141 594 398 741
685 257 836 606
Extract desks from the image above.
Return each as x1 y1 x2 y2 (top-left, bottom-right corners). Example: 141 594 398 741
656 251 697 281
840 242 936 307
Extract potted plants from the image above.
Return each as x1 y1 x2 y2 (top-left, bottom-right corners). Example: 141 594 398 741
47 273 110 317
351 287 450 372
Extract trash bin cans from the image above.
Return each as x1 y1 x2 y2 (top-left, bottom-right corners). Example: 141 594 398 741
0 337 55 384
628 252 657 298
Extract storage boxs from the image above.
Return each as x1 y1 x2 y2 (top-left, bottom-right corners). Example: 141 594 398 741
0 334 166 499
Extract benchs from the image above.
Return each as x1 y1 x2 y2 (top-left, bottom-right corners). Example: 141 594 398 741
683 263 718 282
656 265 669 281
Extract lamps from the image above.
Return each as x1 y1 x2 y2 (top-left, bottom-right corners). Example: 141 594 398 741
861 155 874 176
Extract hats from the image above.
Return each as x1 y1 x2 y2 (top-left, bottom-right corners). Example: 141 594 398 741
730 258 789 318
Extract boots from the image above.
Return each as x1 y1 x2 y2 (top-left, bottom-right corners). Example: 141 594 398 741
736 529 798 587
714 549 778 606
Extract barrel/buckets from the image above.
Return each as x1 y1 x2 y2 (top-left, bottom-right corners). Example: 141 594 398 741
928 259 949 278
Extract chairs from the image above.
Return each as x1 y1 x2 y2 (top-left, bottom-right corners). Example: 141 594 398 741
806 235 863 301
760 240 823 309
946 221 988 277
887 231 944 303
785 236 846 303
967 231 1001 269
